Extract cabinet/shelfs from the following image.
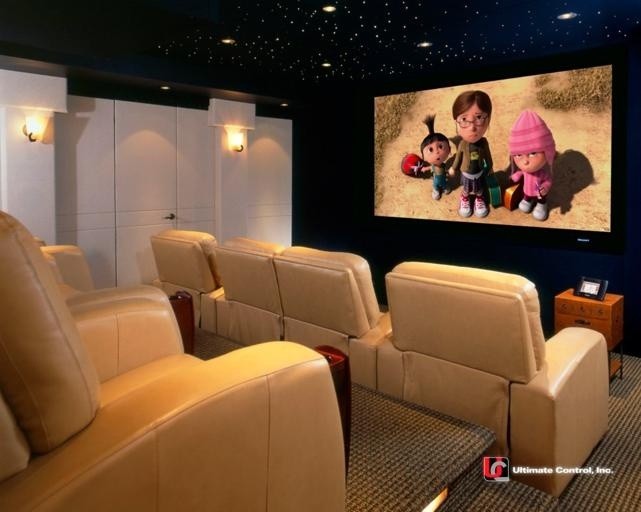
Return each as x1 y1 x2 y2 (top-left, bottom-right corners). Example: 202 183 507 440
554 288 624 380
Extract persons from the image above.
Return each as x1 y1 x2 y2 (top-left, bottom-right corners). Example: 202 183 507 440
420 112 457 200
508 110 556 221
447 90 494 218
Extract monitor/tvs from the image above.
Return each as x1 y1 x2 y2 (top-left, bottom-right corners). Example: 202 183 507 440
364 42 630 256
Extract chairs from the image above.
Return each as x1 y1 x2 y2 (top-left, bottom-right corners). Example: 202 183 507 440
0 210 609 512
